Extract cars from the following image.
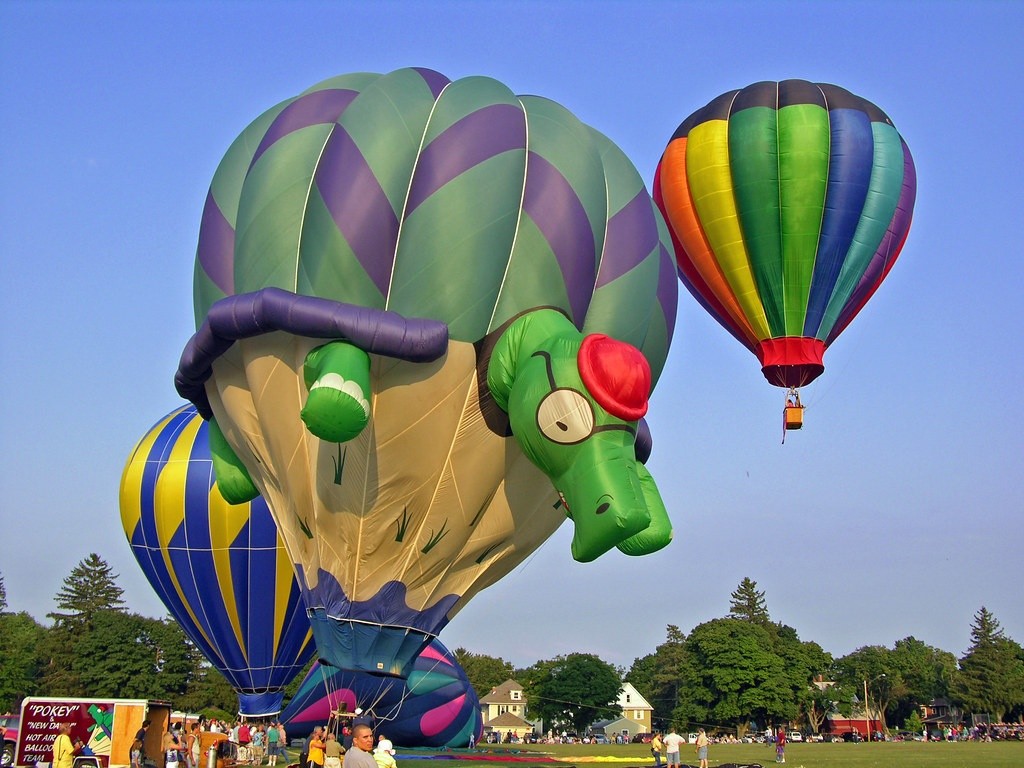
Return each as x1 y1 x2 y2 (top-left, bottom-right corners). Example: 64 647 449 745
824 734 844 743
590 734 606 744
802 732 823 742
864 730 885 742
898 731 924 743
565 731 577 743
839 731 865 743
927 730 943 740
484 730 497 743
523 733 543 743
632 733 653 743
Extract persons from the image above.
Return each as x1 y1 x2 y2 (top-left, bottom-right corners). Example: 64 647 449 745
788 399 794 407
0 727 7 763
128 700 396 768
52 723 83 768
467 722 1024 768
794 397 801 407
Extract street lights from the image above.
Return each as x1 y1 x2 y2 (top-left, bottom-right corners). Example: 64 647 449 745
864 673 886 742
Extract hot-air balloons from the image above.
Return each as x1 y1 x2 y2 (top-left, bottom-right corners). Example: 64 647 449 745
172 64 680 751
117 399 318 732
275 637 485 749
650 78 919 443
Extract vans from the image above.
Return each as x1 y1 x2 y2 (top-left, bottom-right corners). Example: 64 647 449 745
785 731 803 743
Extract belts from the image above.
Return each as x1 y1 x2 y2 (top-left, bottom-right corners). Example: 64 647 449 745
327 756 339 758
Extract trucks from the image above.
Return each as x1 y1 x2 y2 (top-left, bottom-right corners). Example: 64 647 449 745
0 695 231 768
